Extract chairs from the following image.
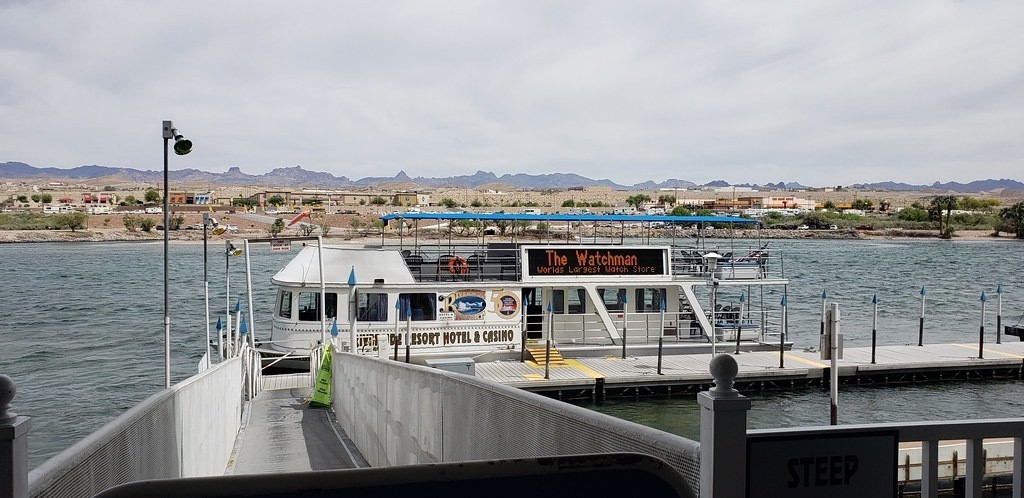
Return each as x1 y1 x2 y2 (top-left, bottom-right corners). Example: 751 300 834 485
500 255 519 281
682 251 732 276
715 304 740 324
465 255 485 282
406 255 423 282
436 255 456 282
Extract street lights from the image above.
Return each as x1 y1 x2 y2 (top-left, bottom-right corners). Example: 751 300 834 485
163 120 193 389
224 239 242 359
203 212 230 369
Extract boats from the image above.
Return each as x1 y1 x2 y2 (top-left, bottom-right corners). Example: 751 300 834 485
210 212 792 368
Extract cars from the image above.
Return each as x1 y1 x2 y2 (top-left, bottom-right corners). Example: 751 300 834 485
830 225 838 230
798 225 809 230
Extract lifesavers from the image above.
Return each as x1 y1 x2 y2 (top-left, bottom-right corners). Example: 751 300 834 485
448 256 467 274
